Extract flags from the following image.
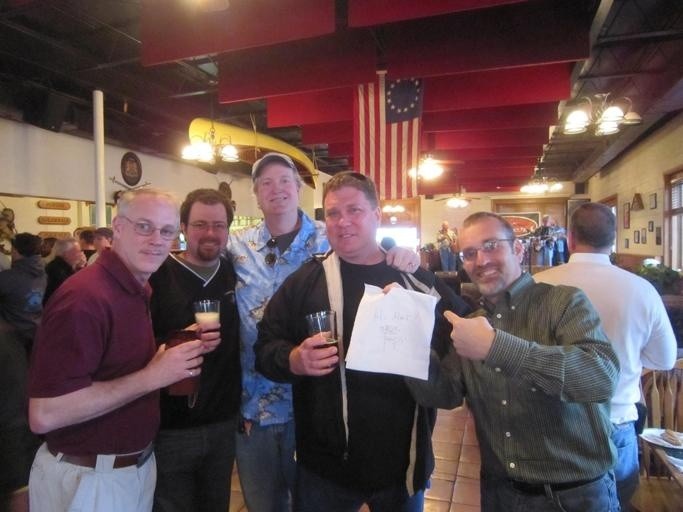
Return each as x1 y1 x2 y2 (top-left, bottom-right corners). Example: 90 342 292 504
349 78 425 200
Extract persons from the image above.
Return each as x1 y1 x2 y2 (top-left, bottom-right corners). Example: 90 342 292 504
1 228 113 501
226 150 421 512
532 215 556 267
529 201 678 512
26 187 206 512
146 186 241 512
435 221 458 274
252 170 475 512
380 211 623 512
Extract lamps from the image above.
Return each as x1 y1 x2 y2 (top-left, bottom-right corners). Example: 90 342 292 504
520 156 562 195
559 90 641 138
180 93 241 164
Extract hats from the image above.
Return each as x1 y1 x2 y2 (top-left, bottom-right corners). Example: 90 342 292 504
252 152 295 181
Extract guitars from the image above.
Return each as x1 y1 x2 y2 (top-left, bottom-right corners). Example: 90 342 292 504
534 227 562 251
439 230 457 253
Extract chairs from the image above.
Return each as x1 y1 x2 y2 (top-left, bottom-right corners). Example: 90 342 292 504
630 361 682 511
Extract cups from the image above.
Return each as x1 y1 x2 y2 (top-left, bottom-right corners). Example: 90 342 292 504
304 311 335 340
192 300 220 324
165 329 201 397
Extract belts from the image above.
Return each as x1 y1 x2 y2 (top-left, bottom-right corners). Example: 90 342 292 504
509 473 606 494
48 441 154 467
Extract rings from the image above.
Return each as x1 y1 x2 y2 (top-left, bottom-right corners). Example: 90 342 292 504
189 369 193 377
407 262 415 267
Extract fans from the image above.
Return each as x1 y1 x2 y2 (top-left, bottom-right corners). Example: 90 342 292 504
434 193 482 202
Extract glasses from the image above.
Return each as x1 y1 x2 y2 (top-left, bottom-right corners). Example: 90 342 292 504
463 238 510 261
123 215 176 240
188 223 229 231
265 237 278 266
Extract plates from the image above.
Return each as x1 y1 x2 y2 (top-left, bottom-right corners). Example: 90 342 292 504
639 428 683 450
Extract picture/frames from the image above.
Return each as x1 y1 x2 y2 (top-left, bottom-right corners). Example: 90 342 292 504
622 192 662 247
